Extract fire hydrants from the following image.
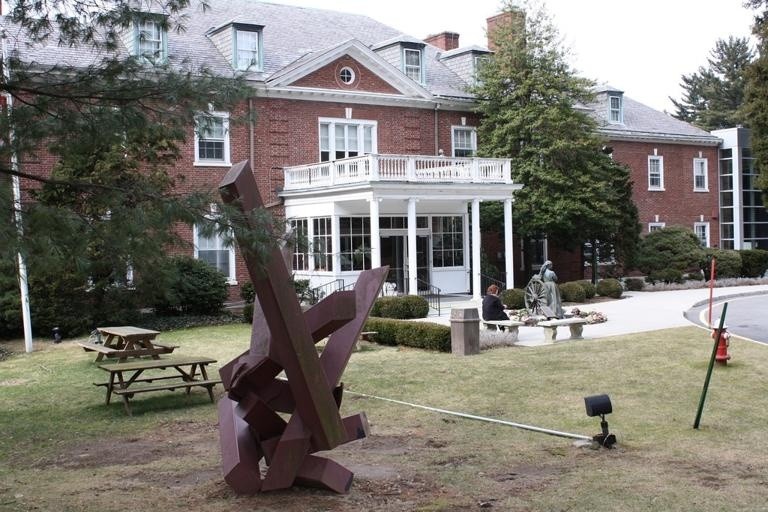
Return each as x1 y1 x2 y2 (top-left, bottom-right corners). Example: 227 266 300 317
708 317 731 364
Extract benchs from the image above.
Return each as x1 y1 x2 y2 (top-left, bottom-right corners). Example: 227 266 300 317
78 337 118 357
480 319 520 340
110 374 225 408
536 317 589 344
149 341 180 356
92 372 199 390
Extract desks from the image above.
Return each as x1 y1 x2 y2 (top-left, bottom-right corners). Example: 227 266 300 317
93 325 167 372
96 355 219 411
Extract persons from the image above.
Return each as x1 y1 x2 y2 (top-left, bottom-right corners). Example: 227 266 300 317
539 260 563 319
482 284 510 331
